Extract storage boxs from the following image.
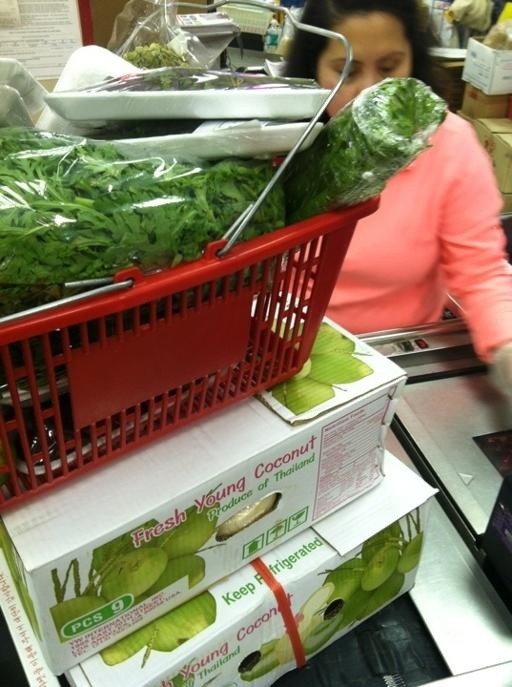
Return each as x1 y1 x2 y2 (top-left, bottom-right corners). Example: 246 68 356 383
428 36 512 216
0 447 440 687
0 292 408 677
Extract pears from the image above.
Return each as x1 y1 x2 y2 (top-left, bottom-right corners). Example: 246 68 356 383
49 504 222 668
266 318 375 417
239 503 425 682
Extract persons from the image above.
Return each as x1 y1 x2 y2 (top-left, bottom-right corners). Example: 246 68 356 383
274 0 511 398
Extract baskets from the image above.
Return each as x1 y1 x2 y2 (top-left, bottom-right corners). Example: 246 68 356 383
0 0 382 514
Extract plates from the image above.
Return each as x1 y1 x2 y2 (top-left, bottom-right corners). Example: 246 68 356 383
81 120 323 169
44 86 331 123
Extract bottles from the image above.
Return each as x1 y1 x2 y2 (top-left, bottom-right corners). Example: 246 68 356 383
266 15 282 50
